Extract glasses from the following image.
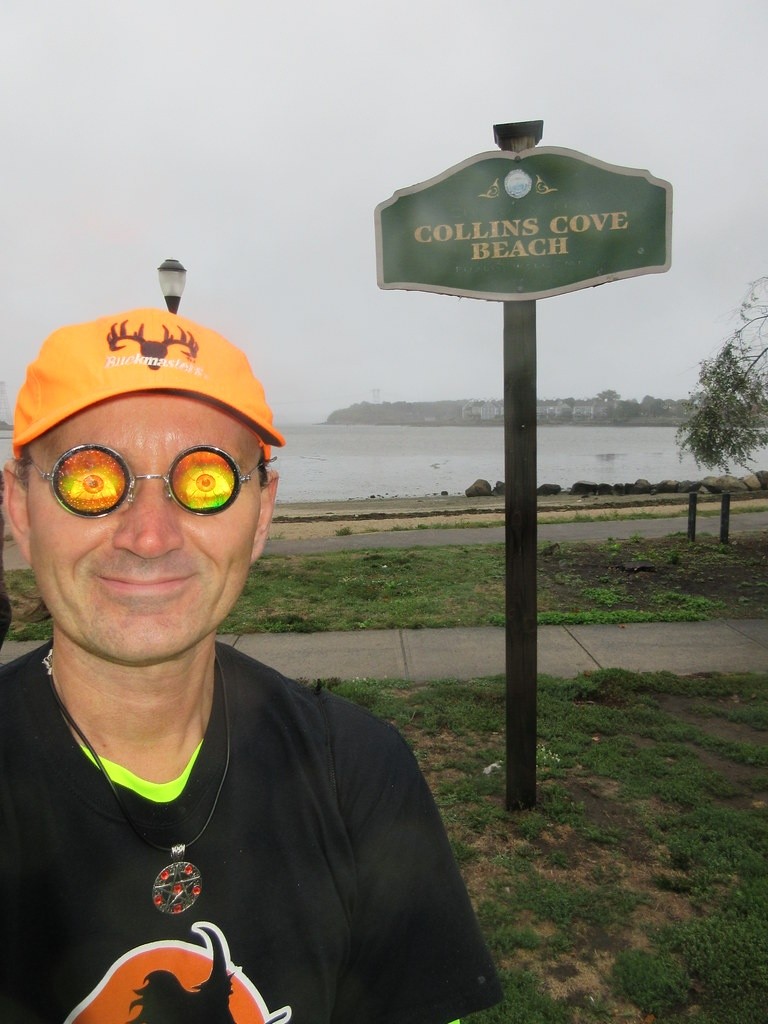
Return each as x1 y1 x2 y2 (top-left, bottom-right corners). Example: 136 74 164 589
19 445 265 518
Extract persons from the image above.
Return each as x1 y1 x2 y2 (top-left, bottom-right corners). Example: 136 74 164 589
0 310 505 1024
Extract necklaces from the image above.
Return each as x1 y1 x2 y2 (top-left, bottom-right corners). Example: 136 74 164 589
41 648 231 913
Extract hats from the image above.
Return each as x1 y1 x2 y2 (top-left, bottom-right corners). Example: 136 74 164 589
13 308 285 465
